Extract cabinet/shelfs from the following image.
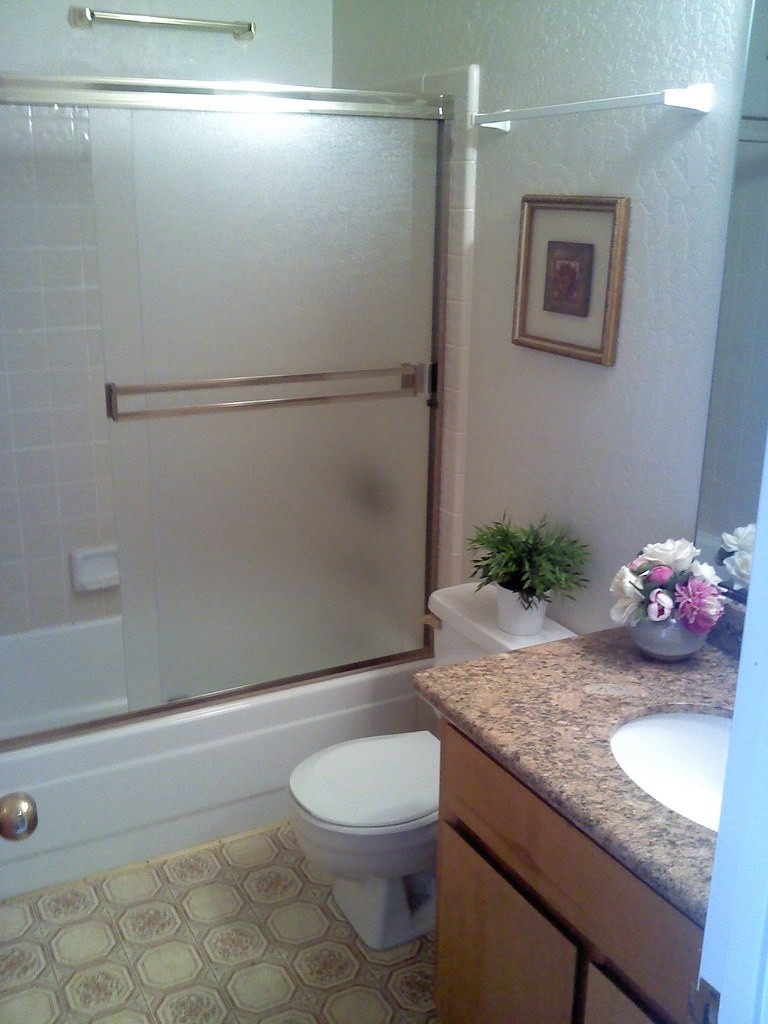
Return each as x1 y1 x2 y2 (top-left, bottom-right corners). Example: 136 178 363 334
432 722 720 1024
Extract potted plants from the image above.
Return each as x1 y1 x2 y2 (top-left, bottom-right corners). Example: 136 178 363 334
465 508 594 637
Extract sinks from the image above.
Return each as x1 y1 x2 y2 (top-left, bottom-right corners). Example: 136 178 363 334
608 701 734 834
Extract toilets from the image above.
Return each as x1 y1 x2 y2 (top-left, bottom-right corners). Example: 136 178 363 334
286 582 583 951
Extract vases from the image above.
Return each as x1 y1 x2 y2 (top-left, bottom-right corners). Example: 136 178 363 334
625 609 710 663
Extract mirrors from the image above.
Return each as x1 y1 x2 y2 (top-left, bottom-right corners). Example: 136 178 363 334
692 1 767 604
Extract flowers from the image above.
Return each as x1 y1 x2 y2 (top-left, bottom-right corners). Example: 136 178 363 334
714 523 756 590
609 536 728 637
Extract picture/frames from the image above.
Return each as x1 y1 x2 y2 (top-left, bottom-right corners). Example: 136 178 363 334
511 194 632 367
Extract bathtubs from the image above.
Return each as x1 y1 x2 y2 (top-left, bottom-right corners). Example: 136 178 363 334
0 614 130 747
